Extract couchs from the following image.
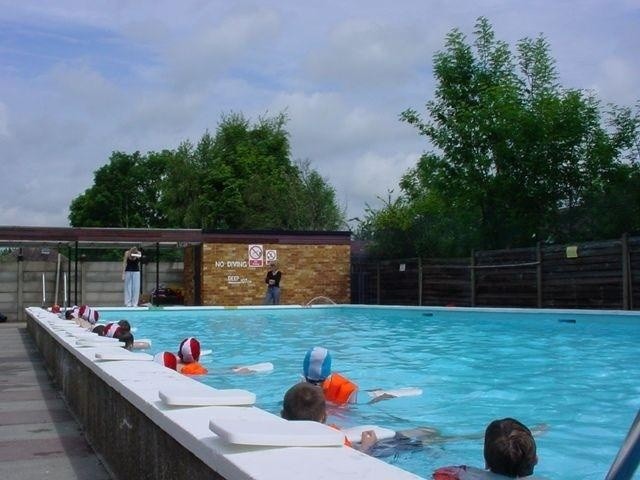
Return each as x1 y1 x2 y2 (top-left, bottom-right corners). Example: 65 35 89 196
150 288 183 305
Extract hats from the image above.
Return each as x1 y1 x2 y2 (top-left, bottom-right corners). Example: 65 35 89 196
103 323 124 339
78 304 100 324
303 346 334 382
179 336 201 362
153 352 176 370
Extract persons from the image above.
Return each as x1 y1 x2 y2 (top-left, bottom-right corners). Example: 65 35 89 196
280 382 548 459
52 301 250 377
121 245 142 308
264 262 281 305
484 417 539 480
